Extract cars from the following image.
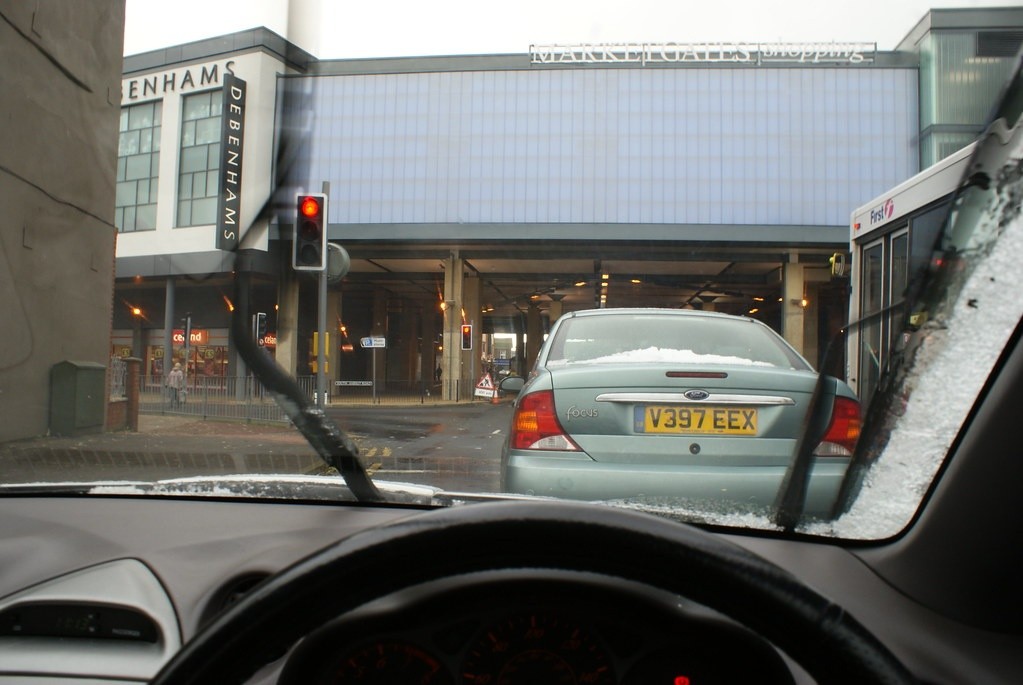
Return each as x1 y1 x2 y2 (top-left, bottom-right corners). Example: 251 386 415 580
497 306 865 528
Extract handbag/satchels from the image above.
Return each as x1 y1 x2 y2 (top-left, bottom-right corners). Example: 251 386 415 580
179 390 188 403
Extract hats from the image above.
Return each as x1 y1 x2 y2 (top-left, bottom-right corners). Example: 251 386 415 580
175 363 181 367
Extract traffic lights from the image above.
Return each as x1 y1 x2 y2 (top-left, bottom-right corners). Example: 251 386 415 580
295 193 329 272
258 312 267 339
460 324 475 351
180 315 188 336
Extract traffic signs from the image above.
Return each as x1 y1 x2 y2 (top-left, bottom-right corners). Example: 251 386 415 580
360 337 386 347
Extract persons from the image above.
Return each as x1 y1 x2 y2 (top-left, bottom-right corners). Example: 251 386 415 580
165 363 183 409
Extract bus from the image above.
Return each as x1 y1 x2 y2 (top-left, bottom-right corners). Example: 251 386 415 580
829 138 982 423
